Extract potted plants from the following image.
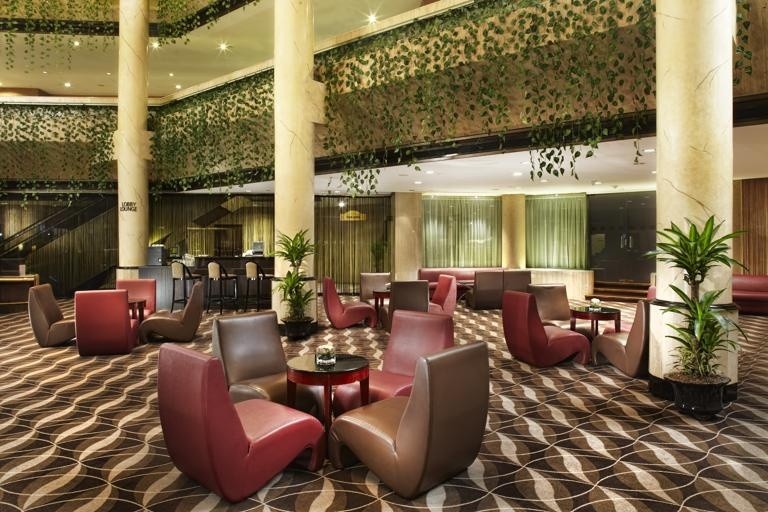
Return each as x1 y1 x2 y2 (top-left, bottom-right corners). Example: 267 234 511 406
268 227 322 342
638 212 751 424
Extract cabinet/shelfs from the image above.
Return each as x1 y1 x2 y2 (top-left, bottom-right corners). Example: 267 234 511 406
0 275 40 305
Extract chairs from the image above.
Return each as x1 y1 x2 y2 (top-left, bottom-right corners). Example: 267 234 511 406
502 290 590 366
170 260 201 314
214 312 325 411
74 290 137 355
117 280 156 320
591 299 651 377
29 283 75 348
158 343 326 503
328 341 488 499
244 260 274 313
321 266 531 330
333 311 453 416
139 279 205 344
529 283 597 339
205 259 240 315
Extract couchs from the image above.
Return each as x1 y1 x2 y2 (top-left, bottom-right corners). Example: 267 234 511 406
732 273 768 315
